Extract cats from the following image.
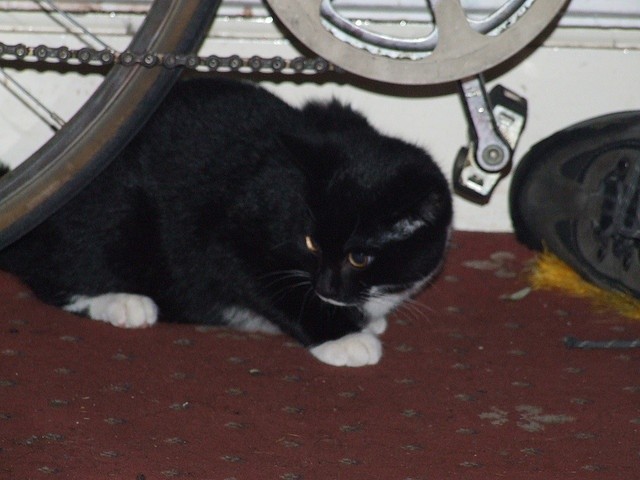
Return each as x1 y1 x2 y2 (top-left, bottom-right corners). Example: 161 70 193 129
0 69 453 367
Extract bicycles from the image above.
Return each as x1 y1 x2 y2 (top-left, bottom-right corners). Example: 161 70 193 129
1 0 570 252
